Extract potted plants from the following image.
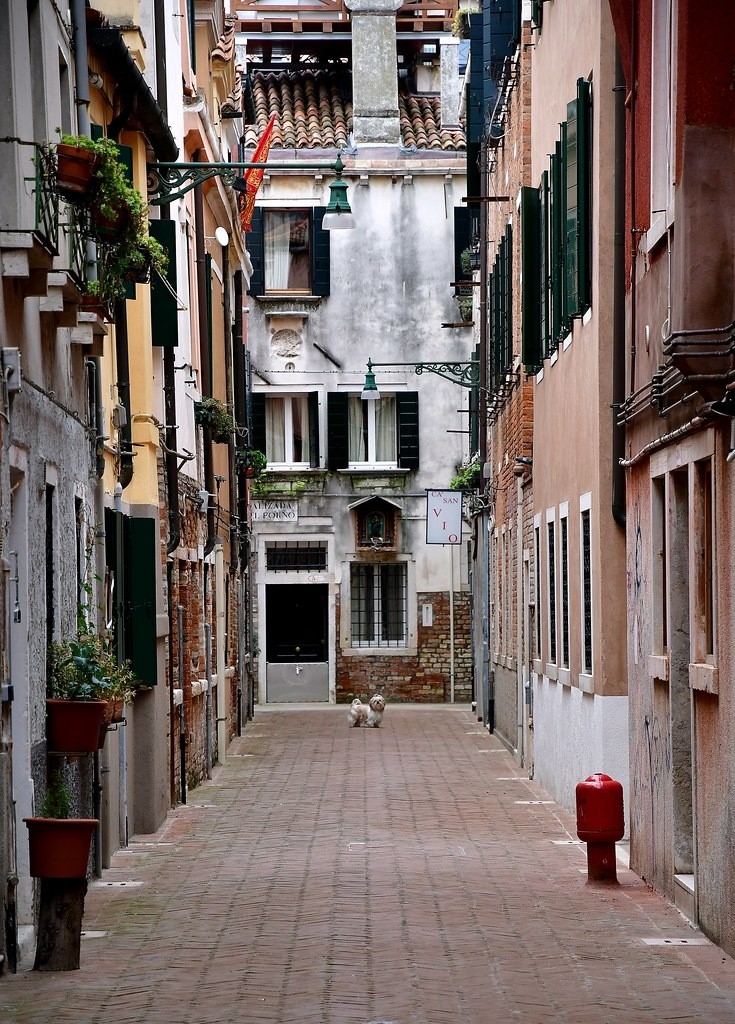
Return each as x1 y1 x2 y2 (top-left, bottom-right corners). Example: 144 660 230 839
35 127 171 322
452 6 480 40
46 501 151 755
234 451 268 477
194 397 237 445
26 757 100 879
460 247 481 269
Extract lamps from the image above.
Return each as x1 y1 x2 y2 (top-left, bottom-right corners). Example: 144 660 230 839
360 358 483 399
148 154 357 230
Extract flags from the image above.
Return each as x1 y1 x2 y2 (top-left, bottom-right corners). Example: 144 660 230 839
240 112 277 233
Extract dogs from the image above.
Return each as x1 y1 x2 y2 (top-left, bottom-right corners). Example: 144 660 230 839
347 694 386 728
